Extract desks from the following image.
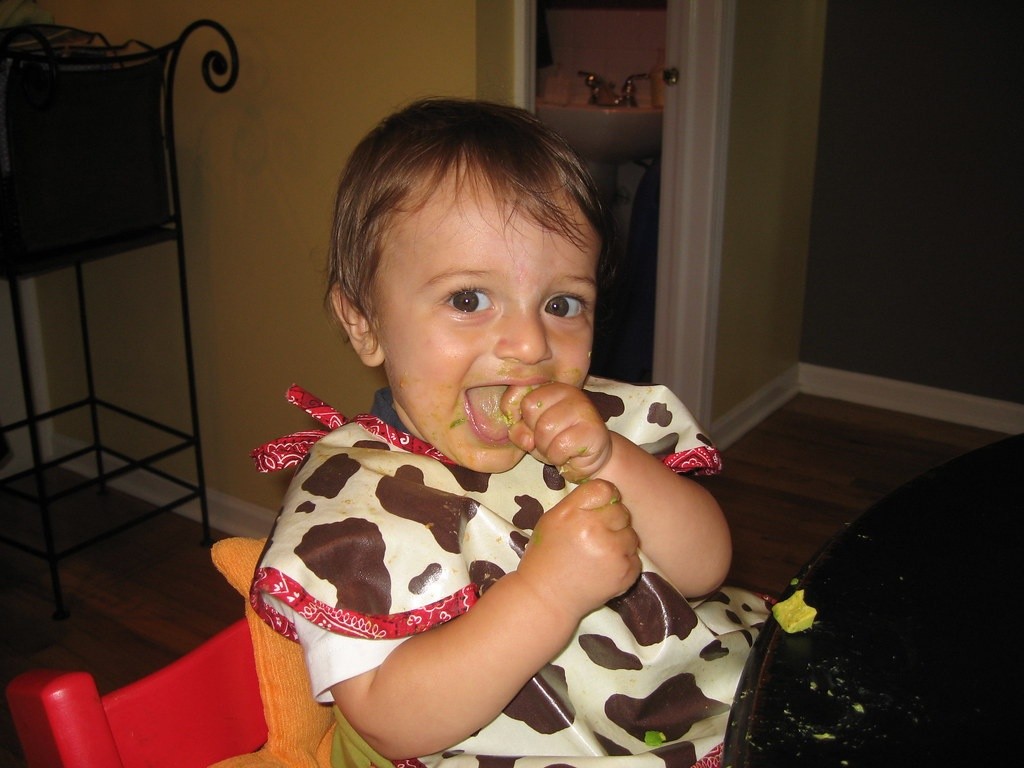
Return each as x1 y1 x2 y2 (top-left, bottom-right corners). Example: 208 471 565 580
723 435 1024 768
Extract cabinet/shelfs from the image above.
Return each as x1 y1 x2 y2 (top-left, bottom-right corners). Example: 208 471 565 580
0 18 239 615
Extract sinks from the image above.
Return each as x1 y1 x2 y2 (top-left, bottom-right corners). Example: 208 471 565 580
534 97 664 161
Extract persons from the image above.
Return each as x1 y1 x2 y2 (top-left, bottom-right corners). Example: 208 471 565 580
250 100 777 768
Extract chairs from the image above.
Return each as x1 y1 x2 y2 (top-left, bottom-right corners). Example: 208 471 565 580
10 616 265 768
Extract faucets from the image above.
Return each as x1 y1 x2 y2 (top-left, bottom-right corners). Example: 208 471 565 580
575 69 650 109
